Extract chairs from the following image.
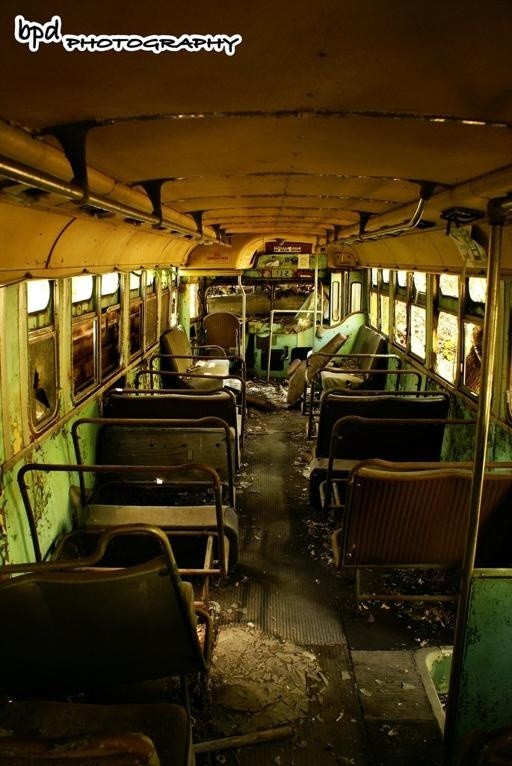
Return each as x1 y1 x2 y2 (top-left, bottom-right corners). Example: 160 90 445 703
0 312 512 765
